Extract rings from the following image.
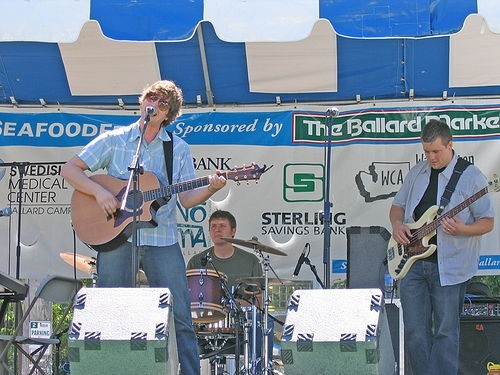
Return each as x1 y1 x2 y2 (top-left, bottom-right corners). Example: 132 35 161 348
448 229 450 231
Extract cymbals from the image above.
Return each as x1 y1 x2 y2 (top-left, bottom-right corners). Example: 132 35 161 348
59 251 146 283
236 274 292 287
220 237 288 256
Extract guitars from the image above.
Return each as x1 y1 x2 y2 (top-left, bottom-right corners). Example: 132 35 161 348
71 163 262 252
386 173 500 281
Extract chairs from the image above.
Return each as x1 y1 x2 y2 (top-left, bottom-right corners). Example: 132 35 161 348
0 276 83 375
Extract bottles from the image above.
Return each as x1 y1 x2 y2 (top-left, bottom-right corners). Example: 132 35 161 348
487 362 500 375
385 267 396 298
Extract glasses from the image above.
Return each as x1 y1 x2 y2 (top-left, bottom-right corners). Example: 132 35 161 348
146 92 170 111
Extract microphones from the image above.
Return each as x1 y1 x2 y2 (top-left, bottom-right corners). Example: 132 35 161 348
326 107 341 117
0 207 13 217
201 251 210 266
145 106 156 114
294 242 308 275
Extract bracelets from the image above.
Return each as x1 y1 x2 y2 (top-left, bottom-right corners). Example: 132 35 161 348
208 186 216 193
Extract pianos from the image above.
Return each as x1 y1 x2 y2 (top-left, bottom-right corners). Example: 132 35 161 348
0 271 30 332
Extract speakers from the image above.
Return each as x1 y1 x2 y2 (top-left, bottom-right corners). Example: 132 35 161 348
280 286 397 375
457 321 500 375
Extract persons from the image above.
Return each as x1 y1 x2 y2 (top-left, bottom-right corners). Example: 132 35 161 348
60 79 227 375
389 119 494 375
188 209 274 375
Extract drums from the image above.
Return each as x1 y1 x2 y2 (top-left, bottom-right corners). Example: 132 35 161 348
194 300 237 339
186 269 227 323
